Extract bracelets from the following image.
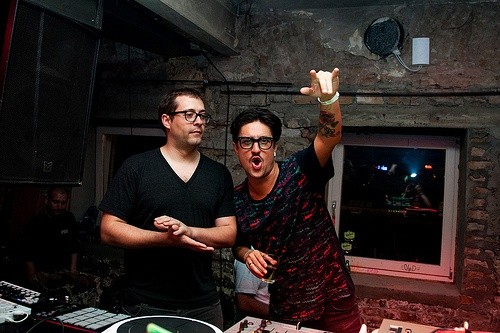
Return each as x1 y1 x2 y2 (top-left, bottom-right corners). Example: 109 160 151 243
318 92 339 105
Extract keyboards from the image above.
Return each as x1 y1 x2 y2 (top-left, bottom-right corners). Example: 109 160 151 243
0 280 59 325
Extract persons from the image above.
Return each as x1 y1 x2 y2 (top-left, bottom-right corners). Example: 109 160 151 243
99 88 237 333
234 259 271 319
232 68 361 333
25 186 79 282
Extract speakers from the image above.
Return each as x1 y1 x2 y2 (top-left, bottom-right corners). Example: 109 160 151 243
0 0 105 187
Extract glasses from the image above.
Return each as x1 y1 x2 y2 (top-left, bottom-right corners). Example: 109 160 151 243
169 111 210 124
236 137 275 150
